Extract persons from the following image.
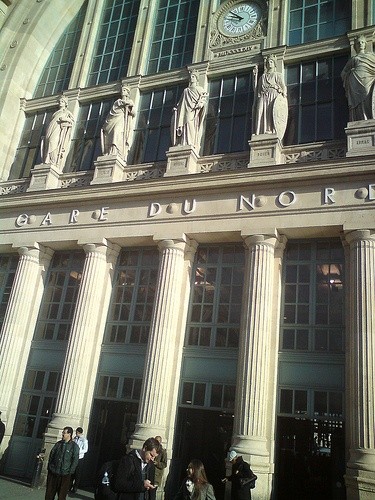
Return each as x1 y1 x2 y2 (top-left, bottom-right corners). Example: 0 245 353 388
341 36 375 121
0 412 5 445
149 436 168 500
252 56 287 136
113 438 161 500
170 72 207 153
45 427 79 500
72 427 88 492
103 87 136 162
41 97 74 167
178 459 216 500
224 451 252 500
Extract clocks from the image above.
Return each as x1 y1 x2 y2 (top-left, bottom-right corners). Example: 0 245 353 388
218 0 262 36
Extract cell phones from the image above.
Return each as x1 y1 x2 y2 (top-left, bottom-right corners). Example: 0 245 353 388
153 485 158 487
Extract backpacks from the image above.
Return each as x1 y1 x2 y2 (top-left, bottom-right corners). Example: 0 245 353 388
94 460 125 500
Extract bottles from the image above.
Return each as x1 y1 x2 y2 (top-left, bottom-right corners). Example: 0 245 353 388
103 477 109 484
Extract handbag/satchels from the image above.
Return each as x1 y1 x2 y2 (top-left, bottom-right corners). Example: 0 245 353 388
239 476 255 488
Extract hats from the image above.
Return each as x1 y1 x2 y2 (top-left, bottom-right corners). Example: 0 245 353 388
226 450 237 462
